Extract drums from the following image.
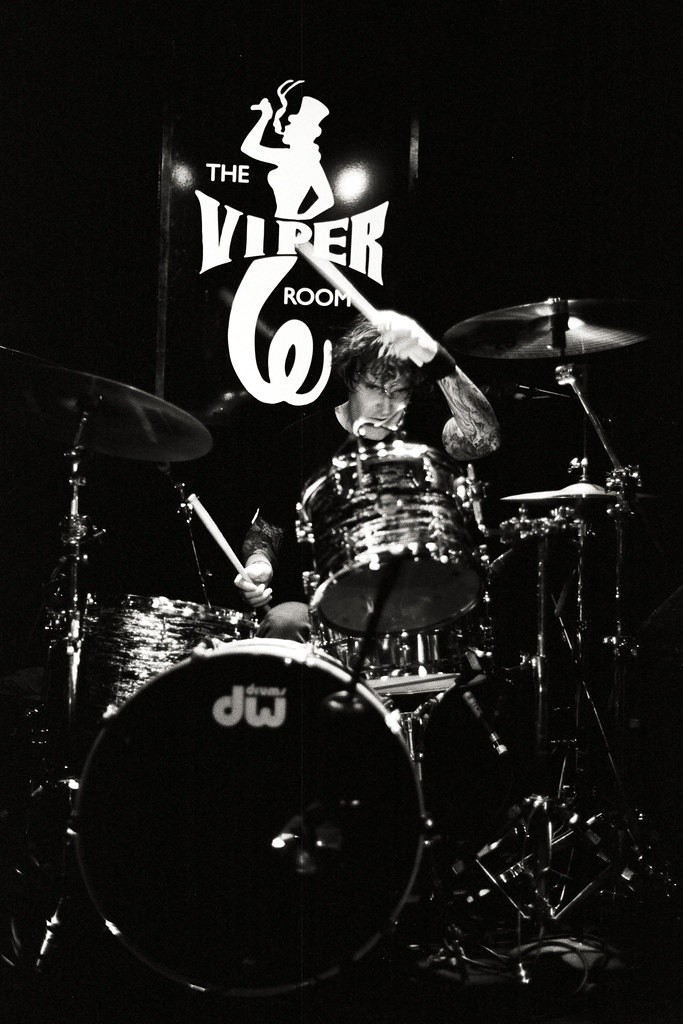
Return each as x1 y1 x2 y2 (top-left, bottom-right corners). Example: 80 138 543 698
96 590 260 725
72 634 434 1001
343 610 485 697
298 439 488 637
616 580 683 900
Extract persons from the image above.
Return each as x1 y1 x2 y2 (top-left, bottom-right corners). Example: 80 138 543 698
234 310 503 644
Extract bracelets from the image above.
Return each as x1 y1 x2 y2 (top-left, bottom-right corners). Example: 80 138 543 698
246 561 270 567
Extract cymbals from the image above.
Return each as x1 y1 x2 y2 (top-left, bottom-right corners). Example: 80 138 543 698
444 289 676 360
1 341 219 466
498 479 660 502
